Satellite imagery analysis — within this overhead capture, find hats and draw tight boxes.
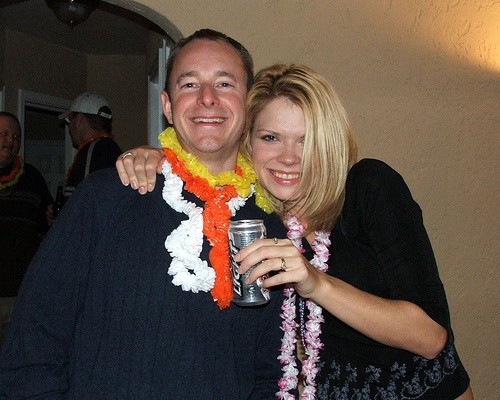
[59,90,112,121]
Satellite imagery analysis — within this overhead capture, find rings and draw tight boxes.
[122,152,134,160]
[280,255,287,271]
[272,237,279,245]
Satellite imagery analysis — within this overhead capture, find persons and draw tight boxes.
[3,28,316,400]
[57,90,123,203]
[1,110,55,298]
[243,62,475,400]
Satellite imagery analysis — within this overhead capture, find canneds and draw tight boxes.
[227,220,270,306]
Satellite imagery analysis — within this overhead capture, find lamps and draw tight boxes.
[44,0,99,27]
